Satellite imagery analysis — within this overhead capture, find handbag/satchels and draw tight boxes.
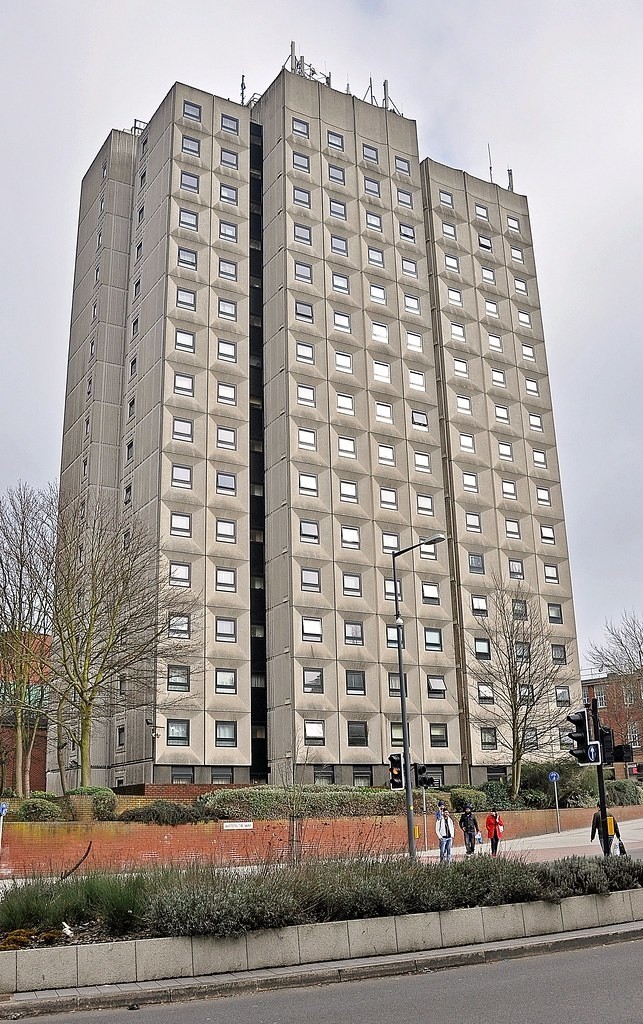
[619,838,626,855]
[475,831,482,843]
[499,826,504,832]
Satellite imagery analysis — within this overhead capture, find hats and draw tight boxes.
[439,802,444,807]
[465,803,471,808]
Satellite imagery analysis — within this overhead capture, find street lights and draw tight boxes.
[392,531,446,863]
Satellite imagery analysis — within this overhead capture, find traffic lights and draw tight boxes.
[389,753,404,791]
[417,765,434,788]
[565,709,592,766]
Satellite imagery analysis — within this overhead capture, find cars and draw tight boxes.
[637,762,643,781]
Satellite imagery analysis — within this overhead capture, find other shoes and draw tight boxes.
[466,850,474,854]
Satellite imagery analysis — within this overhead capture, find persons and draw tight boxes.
[435,809,454,864]
[459,804,479,855]
[590,801,621,855]
[485,809,504,856]
